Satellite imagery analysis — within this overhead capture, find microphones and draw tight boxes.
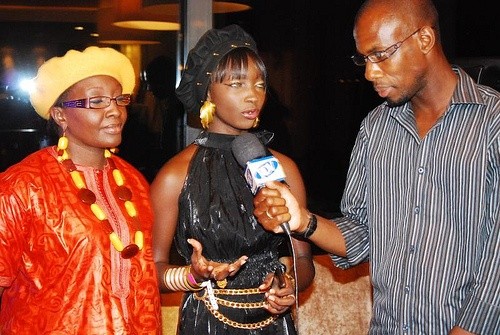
[231,133,291,234]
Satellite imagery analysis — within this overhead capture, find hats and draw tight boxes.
[28,46,135,120]
[176,24,259,116]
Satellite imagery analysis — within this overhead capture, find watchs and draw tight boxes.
[290,213,317,241]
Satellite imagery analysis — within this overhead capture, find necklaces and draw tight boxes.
[54,144,143,259]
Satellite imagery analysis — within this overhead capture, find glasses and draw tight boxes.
[57,94,131,110]
[352,27,437,66]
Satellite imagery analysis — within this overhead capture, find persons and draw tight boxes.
[0,46,163,335]
[252,0,500,335]
[148,25,315,335]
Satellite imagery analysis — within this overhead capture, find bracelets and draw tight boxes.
[162,264,206,293]
[285,270,296,295]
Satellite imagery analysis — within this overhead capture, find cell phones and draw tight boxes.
[269,268,286,298]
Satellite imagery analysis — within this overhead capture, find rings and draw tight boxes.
[214,274,227,288]
[288,294,295,299]
[265,209,273,219]
